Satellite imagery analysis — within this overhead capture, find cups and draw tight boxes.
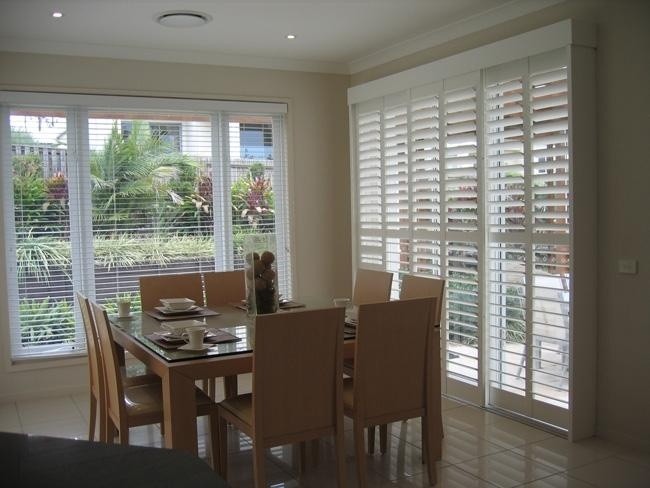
[117,298,130,316]
[181,326,205,348]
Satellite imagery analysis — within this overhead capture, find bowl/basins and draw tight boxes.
[160,319,208,335]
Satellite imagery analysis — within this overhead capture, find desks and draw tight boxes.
[102,298,359,458]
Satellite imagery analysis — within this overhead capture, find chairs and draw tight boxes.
[204,267,249,409]
[138,270,208,396]
[90,301,219,483]
[343,275,445,454]
[214,304,347,486]
[311,294,440,488]
[76,289,163,442]
[340,266,393,305]
[511,283,569,391]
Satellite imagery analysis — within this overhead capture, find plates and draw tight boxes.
[152,329,217,342]
[178,342,214,350]
[159,297,196,309]
[153,304,204,314]
